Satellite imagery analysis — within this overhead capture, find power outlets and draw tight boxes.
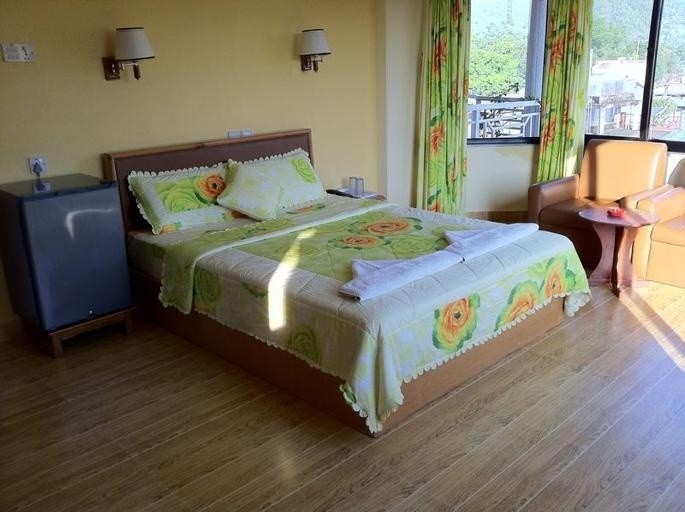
[27,153,46,173]
[2,44,36,64]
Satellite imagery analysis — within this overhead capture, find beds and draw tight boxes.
[103,129,591,438]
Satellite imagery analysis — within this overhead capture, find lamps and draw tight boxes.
[298,27,332,72]
[102,27,155,80]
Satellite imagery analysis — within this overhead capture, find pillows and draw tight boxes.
[218,164,283,221]
[227,147,327,211]
[128,163,227,235]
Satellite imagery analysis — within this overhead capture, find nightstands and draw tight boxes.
[0,174,133,355]
[327,186,384,200]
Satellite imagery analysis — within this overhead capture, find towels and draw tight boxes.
[443,222,539,263]
[337,249,464,303]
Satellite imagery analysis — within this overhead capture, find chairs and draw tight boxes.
[528,139,668,268]
[635,157,685,288]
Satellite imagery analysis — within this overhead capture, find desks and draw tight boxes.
[579,206,660,298]
